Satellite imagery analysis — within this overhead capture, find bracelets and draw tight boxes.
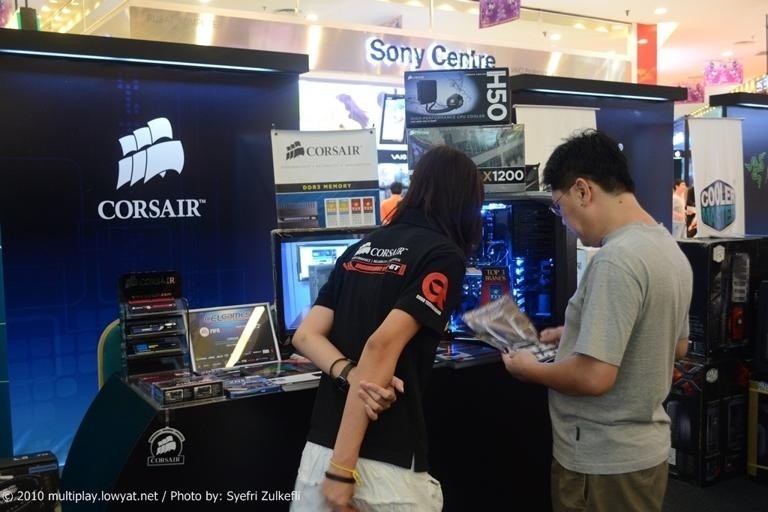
[328,354,349,383]
[324,472,357,485]
[329,459,363,487]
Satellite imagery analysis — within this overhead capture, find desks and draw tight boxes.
[435,336,553,511]
[117,358,446,512]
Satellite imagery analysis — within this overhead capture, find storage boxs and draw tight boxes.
[0,451,58,512]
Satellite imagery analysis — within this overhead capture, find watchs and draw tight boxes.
[335,360,355,390]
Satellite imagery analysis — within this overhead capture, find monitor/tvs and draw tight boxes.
[270,225,382,346]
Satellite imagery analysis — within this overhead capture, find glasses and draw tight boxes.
[548,188,574,215]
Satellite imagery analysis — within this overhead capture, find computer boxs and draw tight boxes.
[446,191,580,341]
[662,235,768,489]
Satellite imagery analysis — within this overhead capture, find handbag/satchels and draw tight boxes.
[288,441,444,511]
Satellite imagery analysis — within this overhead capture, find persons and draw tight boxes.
[673,179,688,240]
[685,186,697,238]
[381,181,404,225]
[288,146,485,511]
[502,128,694,512]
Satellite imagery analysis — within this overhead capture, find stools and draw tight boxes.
[746,387,768,477]
